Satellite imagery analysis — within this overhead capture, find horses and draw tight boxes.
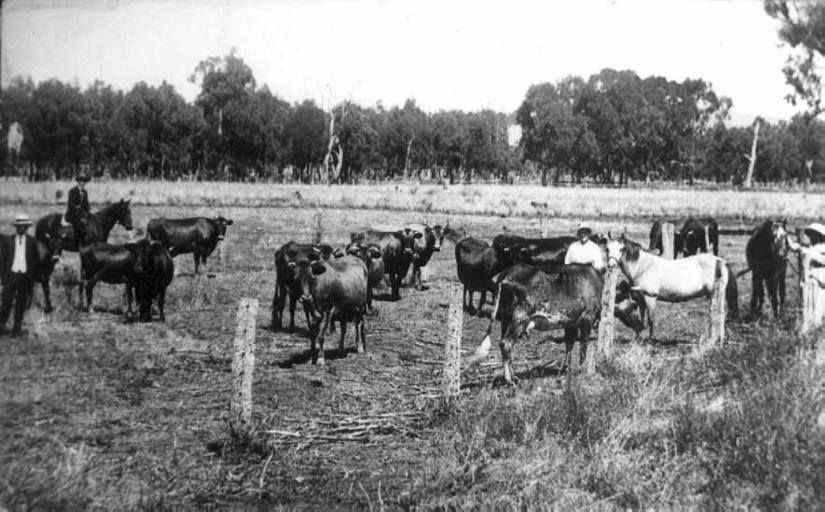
[744,216,791,316]
[602,231,740,342]
[34,197,135,269]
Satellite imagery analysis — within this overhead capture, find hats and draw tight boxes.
[805,223,825,241]
[75,173,90,181]
[13,215,32,225]
[577,223,592,235]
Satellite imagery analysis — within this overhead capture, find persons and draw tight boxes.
[0,214,39,337]
[787,222,825,330]
[64,172,90,236]
[564,228,601,270]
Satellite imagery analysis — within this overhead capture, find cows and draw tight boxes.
[1,230,71,312]
[493,229,605,264]
[453,229,518,315]
[483,261,647,386]
[144,215,234,273]
[648,215,694,256]
[682,215,720,256]
[268,218,446,329]
[288,252,377,366]
[123,235,173,325]
[72,223,176,314]
